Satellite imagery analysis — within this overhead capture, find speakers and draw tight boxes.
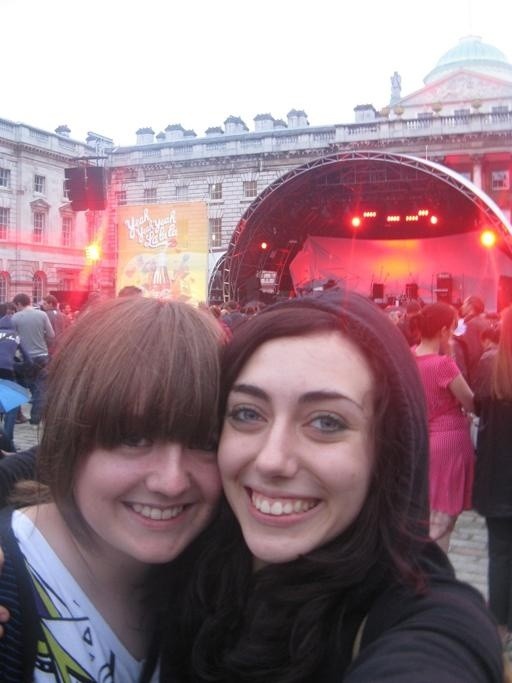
[86,168,108,211]
[63,167,88,212]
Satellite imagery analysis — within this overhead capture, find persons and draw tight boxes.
[0,281,512,683]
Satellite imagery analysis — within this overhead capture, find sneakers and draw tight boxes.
[14,411,41,428]
[1,437,16,454]
